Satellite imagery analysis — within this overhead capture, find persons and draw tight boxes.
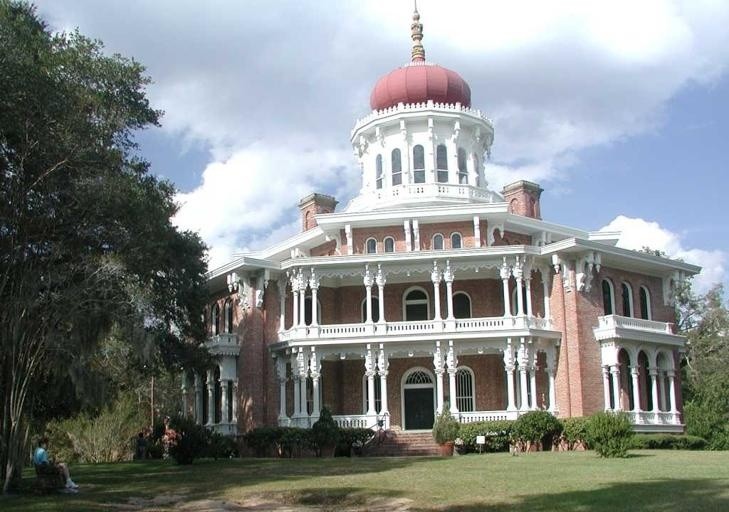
[135,432,152,458]
[33,436,79,495]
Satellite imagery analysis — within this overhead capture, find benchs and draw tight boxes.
[34,462,64,492]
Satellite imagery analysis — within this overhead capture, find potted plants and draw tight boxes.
[247,427,275,458]
[313,404,340,457]
[432,401,459,457]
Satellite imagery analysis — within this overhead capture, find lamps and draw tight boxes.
[340,353,346,360]
[408,351,414,357]
[478,347,483,354]
